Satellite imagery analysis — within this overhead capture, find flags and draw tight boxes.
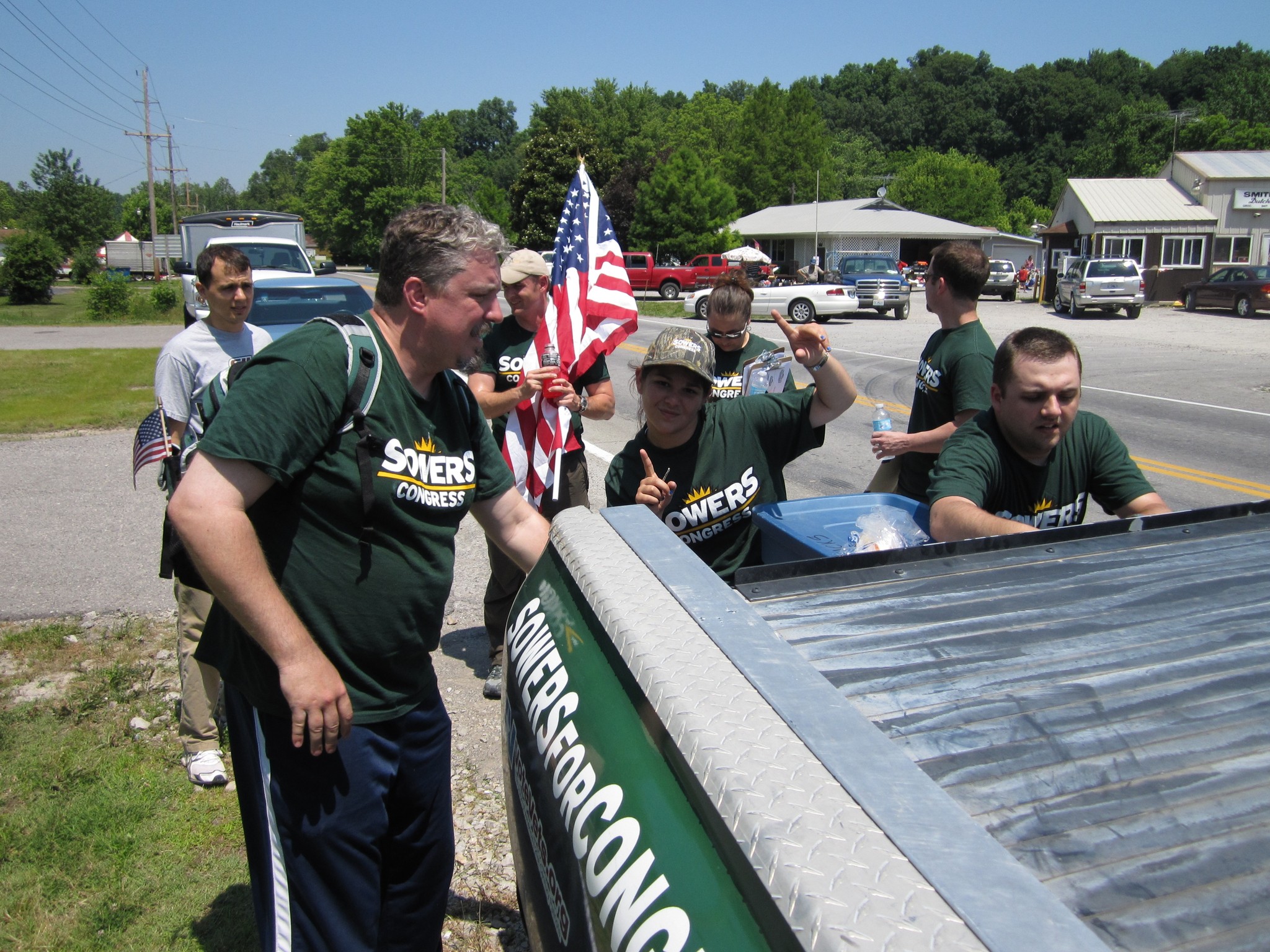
[755,240,762,251]
[501,162,639,514]
[133,407,172,491]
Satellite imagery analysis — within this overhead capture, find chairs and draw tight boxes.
[877,265,887,270]
[1233,275,1245,281]
[846,265,856,272]
[274,252,291,265]
[770,279,790,287]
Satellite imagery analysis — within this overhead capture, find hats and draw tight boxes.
[641,326,716,384]
[810,259,816,264]
[500,248,549,284]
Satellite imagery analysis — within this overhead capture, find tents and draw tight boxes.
[96,231,140,264]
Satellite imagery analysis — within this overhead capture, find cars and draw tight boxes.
[1176,265,1270,319]
[247,278,374,341]
[684,274,859,324]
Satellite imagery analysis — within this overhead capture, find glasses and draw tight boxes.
[706,319,748,338]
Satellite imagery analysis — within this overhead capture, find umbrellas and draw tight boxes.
[721,245,772,268]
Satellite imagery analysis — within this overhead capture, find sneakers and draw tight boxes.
[482,666,503,695]
[184,748,227,784]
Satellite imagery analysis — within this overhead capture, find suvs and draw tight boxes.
[539,251,556,280]
[981,259,1019,301]
[1052,254,1145,319]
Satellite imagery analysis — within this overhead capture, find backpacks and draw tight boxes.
[160,313,384,593]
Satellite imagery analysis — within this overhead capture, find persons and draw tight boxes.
[155,243,274,787]
[896,259,908,275]
[925,327,1174,542]
[863,239,997,503]
[604,310,857,584]
[169,203,552,952]
[703,269,797,402]
[797,259,831,283]
[468,249,615,698]
[1018,255,1034,293]
[741,260,764,275]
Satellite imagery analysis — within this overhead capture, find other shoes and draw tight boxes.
[1016,290,1019,293]
[1024,291,1028,293]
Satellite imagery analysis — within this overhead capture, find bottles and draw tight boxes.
[747,371,769,396]
[541,343,563,398]
[872,403,895,460]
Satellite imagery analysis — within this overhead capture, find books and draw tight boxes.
[742,347,792,396]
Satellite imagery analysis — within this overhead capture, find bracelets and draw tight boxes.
[803,352,829,372]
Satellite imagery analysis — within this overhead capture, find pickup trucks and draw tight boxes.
[504,501,1270,952]
[622,252,697,300]
[824,255,911,319]
[680,254,770,292]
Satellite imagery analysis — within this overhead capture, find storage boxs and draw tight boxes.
[751,492,931,565]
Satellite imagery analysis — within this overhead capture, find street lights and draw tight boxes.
[137,208,144,281]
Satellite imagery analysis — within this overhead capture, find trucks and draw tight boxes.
[174,210,337,329]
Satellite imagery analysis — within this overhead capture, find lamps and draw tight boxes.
[1190,178,1204,196]
[1031,219,1048,233]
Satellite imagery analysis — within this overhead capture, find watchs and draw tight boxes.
[579,395,588,414]
